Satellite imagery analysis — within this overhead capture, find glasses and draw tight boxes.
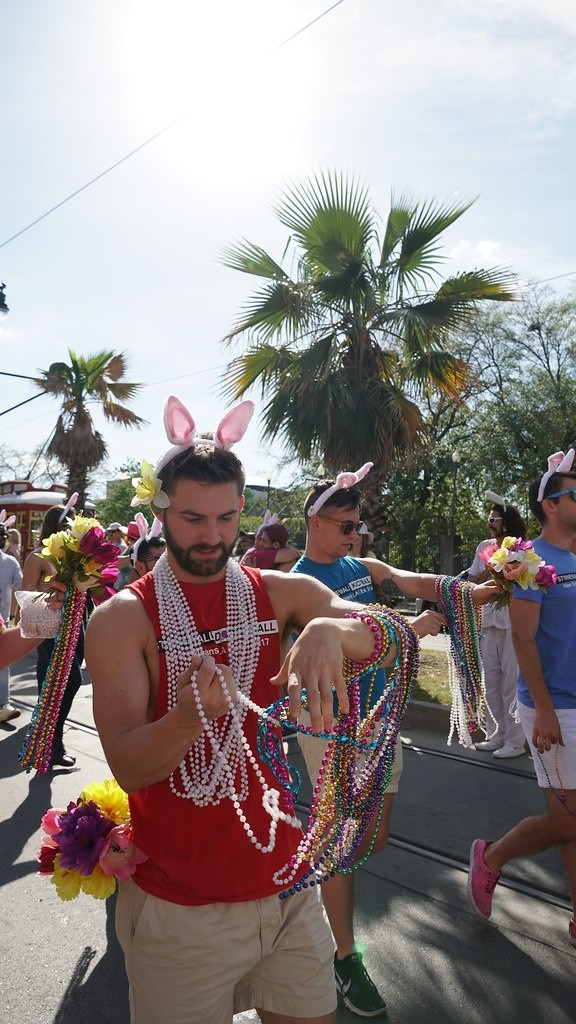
[487,517,503,525]
[546,489,576,501]
[319,516,364,535]
[144,555,161,561]
[108,530,116,533]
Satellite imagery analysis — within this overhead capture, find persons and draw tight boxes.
[276,480,504,1017]
[0,505,377,764]
[467,448,576,945]
[83,437,406,1024]
[465,488,526,758]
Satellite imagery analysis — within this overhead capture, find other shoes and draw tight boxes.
[51,754,75,766]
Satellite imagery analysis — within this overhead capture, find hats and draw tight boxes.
[266,525,288,542]
[127,524,140,541]
[106,522,122,530]
[358,521,374,545]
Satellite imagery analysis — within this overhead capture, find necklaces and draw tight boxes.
[434,574,520,749]
[17,586,87,775]
[193,603,419,896]
[537,740,566,805]
[155,546,259,804]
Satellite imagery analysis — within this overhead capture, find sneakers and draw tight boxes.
[0,704,21,724]
[475,741,502,750]
[334,950,388,1017]
[568,919,576,946]
[468,839,502,919]
[494,747,526,758]
